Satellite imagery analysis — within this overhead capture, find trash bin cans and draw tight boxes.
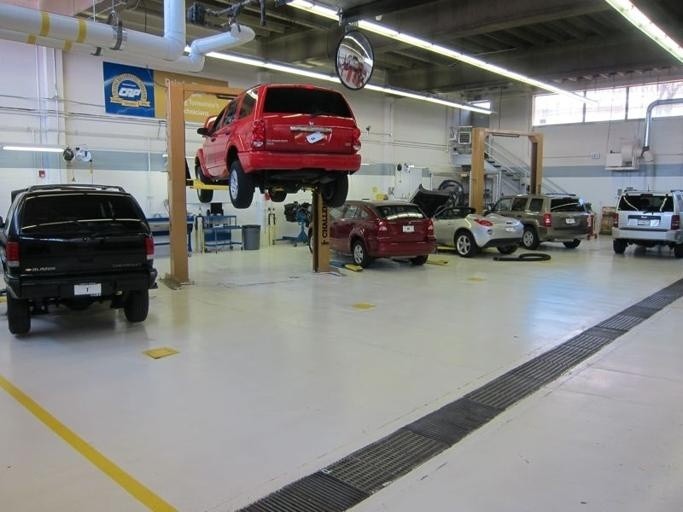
[242,224,261,250]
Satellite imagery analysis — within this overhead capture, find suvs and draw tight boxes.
[487,193,595,250]
[610,188,682,258]
[1,182,159,335]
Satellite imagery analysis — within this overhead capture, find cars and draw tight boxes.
[429,203,525,258]
[191,82,361,210]
[307,200,438,269]
